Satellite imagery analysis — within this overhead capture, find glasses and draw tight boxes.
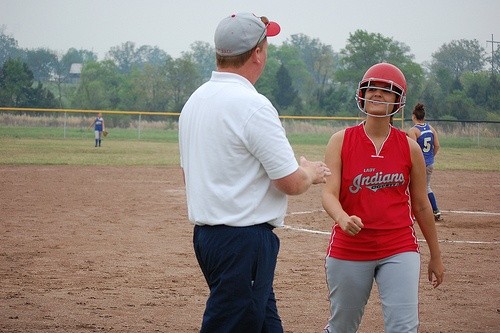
[256,16,270,47]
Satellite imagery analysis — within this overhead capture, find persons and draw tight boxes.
[407,104,441,222]
[176,10,333,333]
[320,62,446,333]
[89,111,105,147]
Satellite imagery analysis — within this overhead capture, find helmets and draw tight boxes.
[354,63,406,117]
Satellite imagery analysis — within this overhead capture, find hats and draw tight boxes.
[214,12,280,56]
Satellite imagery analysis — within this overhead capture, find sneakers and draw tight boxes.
[434,209,441,220]
